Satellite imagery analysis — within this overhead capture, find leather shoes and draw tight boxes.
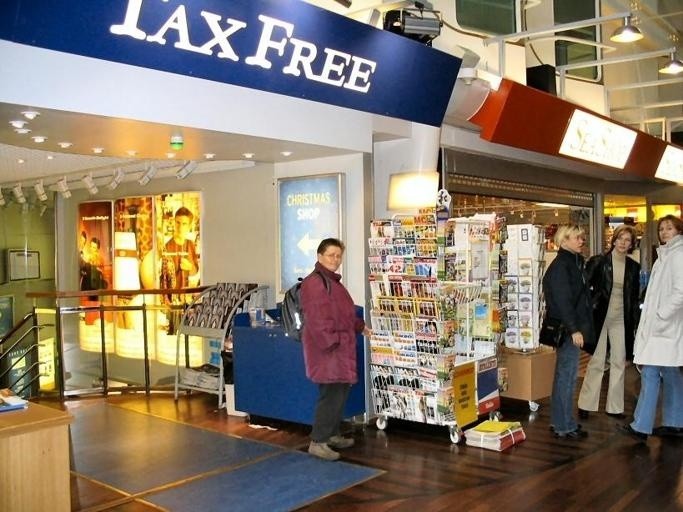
[605,412,627,421]
[653,425,682,436]
[549,423,591,442]
[615,423,648,443]
[576,407,590,420]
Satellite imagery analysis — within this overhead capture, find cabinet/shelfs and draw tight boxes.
[167,278,275,412]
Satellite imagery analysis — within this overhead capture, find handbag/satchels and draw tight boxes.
[539,282,585,351]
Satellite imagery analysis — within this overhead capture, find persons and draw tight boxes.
[614,214,683,443]
[543,225,596,440]
[578,225,641,420]
[159,207,200,321]
[80,231,104,273]
[300,238,373,462]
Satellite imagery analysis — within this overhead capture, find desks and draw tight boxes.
[230,303,366,433]
[0,386,75,511]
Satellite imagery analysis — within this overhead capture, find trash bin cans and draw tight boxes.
[221,350,248,417]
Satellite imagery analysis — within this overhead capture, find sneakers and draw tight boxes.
[326,434,355,450]
[306,441,341,462]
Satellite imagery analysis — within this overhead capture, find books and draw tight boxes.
[368,207,491,424]
[464,419,526,452]
[0,388,28,412]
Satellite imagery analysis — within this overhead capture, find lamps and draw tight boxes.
[451,194,559,222]
[0,156,197,210]
[555,45,683,104]
[385,168,441,211]
[479,9,647,82]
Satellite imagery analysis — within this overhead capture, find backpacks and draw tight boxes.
[279,269,331,344]
